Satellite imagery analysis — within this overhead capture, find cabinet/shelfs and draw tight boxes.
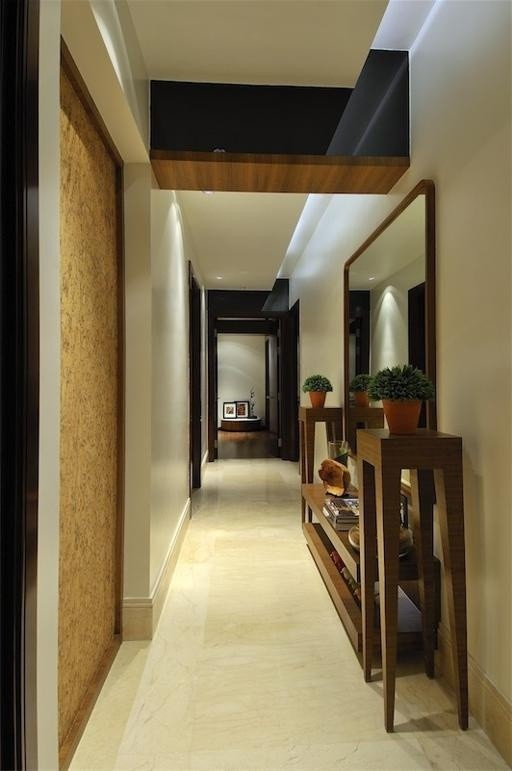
[220,418,263,431]
[345,405,382,455]
[301,478,442,659]
[349,421,472,732]
[298,407,344,532]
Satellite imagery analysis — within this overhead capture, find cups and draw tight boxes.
[327,441,349,469]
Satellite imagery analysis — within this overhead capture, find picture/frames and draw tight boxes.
[234,401,251,419]
[222,402,238,420]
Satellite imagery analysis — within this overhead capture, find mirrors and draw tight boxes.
[342,179,438,460]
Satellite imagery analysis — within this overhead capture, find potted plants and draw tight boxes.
[349,373,374,407]
[301,375,334,410]
[368,363,435,439]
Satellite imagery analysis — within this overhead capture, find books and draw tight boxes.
[320,496,360,532]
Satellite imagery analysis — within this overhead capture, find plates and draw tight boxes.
[348,524,414,562]
